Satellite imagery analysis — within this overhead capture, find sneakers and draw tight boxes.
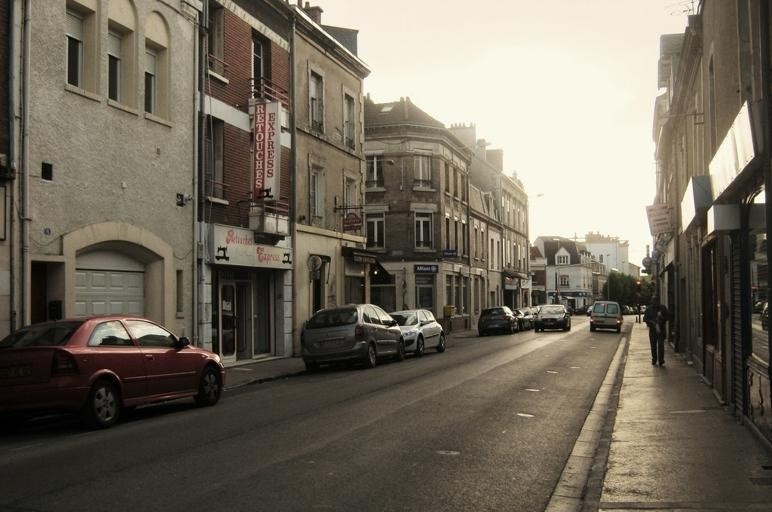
[651,358,666,366]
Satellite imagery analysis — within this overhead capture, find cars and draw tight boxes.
[622,305,646,314]
[478,305,573,337]
[0,314,226,429]
[301,303,446,374]
[587,306,594,316]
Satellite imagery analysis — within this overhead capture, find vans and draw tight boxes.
[590,300,623,333]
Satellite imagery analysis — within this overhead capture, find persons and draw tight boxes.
[643,294,671,368]
